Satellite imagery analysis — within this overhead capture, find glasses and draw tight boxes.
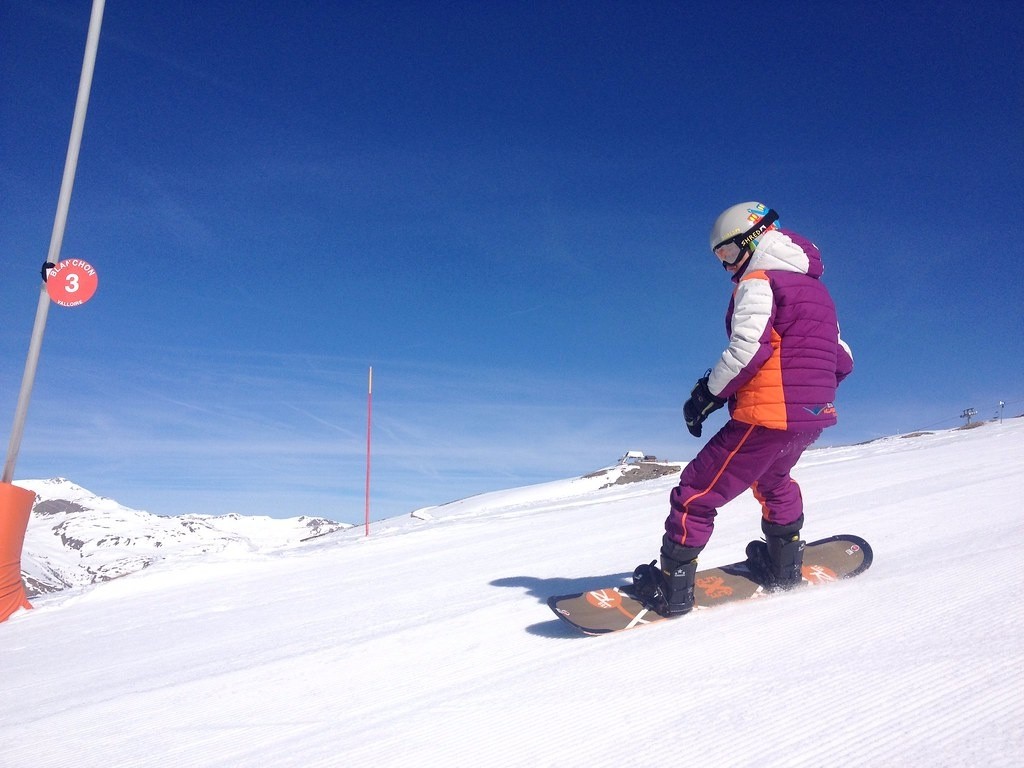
[713,209,779,271]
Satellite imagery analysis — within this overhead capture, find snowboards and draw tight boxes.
[549,534,874,634]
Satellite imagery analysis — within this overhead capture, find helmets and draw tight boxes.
[710,202,781,252]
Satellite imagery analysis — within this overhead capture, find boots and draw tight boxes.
[633,555,698,617]
[746,530,817,591]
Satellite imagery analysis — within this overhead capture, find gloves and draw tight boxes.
[683,368,728,437]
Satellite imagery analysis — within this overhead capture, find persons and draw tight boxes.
[634,201,854,616]
